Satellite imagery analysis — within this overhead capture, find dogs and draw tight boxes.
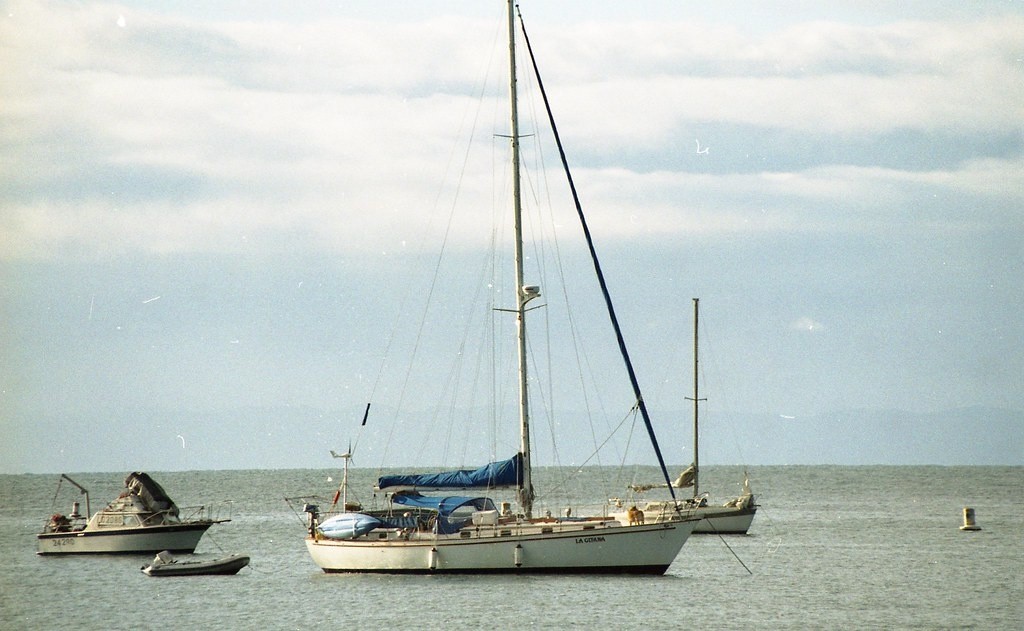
[627,506,645,526]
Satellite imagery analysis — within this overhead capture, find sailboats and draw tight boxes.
[606,297,761,535]
[284,0,707,576]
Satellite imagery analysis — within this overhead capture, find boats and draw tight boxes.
[34,470,237,556]
[139,546,250,578]
